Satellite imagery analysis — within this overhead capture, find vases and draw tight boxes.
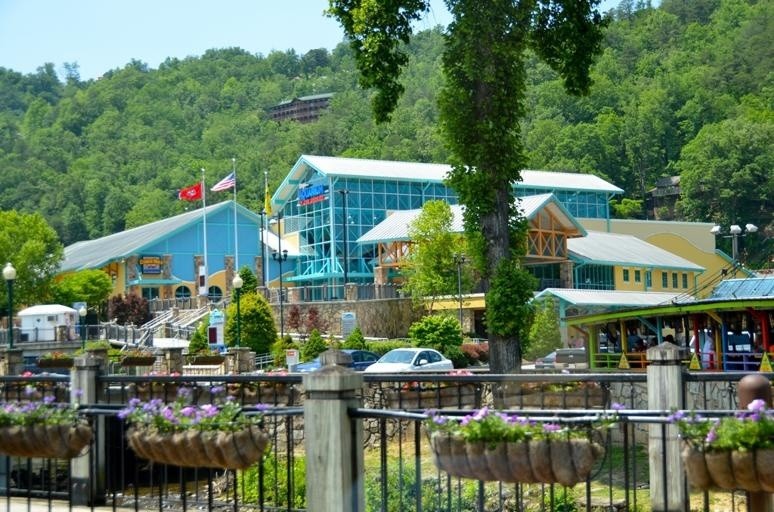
[427,431,608,489]
[126,427,271,469]
[678,434,774,493]
[0,424,95,459]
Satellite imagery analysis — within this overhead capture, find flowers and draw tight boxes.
[0,384,84,425]
[420,399,631,440]
[665,399,774,451]
[117,384,267,431]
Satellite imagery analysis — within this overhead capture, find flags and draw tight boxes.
[210,171,235,192]
[265,181,273,217]
[179,182,202,200]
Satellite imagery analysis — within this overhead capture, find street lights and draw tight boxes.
[271,208,289,338]
[78,306,86,352]
[232,274,243,348]
[452,251,466,338]
[338,187,352,288]
[2,262,16,348]
[710,222,758,279]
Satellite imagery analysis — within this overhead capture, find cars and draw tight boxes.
[364,347,453,390]
[535,329,757,370]
[294,348,379,377]
